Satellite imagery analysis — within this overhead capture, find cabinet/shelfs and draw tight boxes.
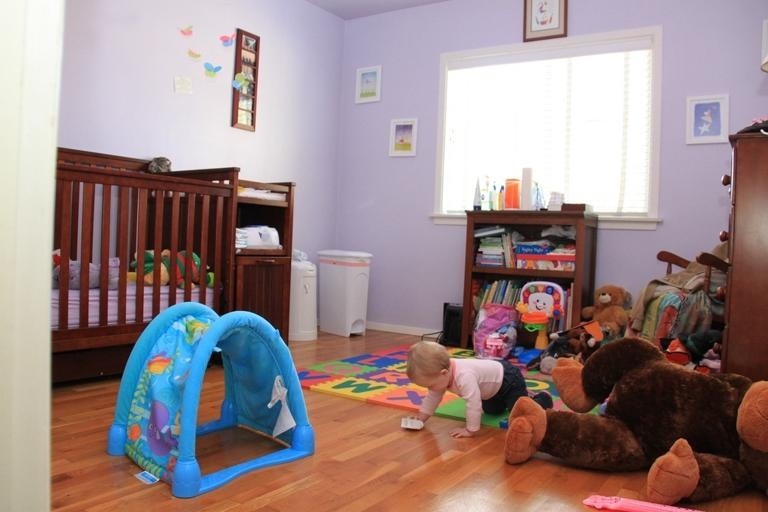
[460,209,598,349]
[161,167,296,346]
[717,130,768,383]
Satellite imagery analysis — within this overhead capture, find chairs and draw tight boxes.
[656,248,729,365]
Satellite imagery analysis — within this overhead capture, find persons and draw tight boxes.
[406,341,551,438]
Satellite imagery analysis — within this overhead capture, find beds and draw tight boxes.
[49,146,240,356]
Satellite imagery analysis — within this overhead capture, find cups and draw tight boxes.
[504,179,521,209]
[521,167,533,211]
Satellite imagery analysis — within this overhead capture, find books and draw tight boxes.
[472,228,573,329]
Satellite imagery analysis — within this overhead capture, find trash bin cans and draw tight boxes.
[288,249,374,342]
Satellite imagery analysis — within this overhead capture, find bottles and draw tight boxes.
[473,175,504,210]
[533,183,546,210]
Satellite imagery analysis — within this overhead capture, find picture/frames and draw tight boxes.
[232,27,260,132]
[523,0,568,42]
[685,94,729,144]
[354,64,381,104]
[388,118,417,157]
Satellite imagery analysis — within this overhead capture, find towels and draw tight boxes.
[234,223,280,249]
